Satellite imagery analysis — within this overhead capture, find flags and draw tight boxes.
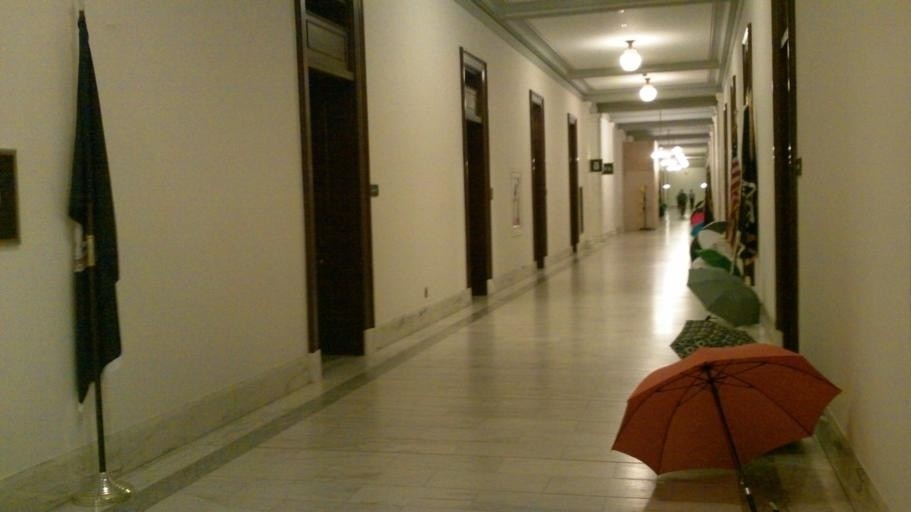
[62,12,128,402]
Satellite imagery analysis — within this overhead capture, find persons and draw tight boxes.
[688,190,695,209]
[676,189,688,217]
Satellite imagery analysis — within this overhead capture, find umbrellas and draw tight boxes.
[685,267,762,328]
[609,341,845,512]
[690,200,746,280]
[669,319,755,366]
